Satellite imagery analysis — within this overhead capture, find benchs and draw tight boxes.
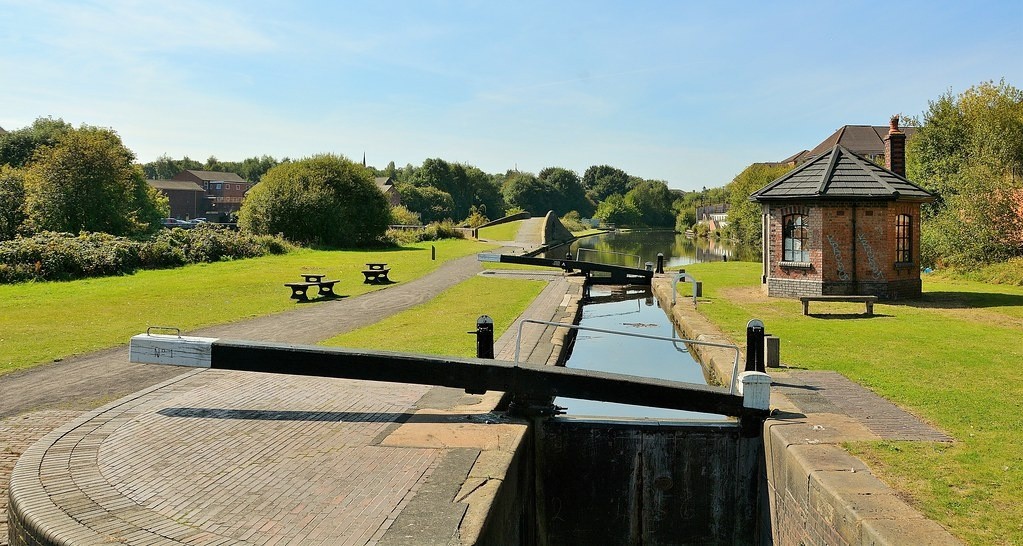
[283,280,340,301]
[361,267,391,284]
[797,295,879,316]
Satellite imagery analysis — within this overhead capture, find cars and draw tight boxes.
[161,218,206,229]
